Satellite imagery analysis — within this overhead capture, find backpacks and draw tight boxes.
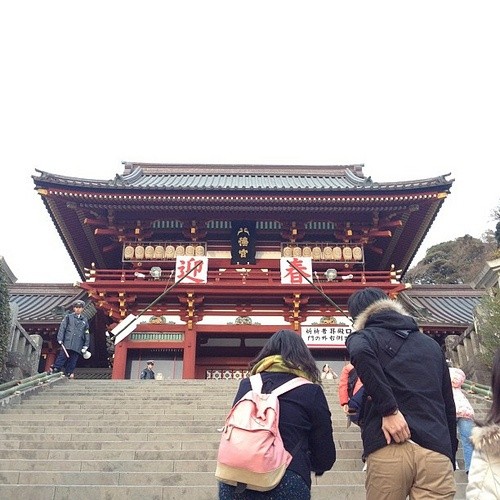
[214,372,315,491]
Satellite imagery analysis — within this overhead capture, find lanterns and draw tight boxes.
[135,246,144,259]
[303,246,321,261]
[343,247,362,261]
[186,246,204,256]
[125,246,134,259]
[155,246,164,259]
[165,245,185,260]
[323,246,343,261]
[145,246,154,259]
[283,247,301,257]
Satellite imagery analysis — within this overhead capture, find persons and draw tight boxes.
[340,362,363,413]
[345,287,459,500]
[448,367,477,474]
[465,346,500,500]
[220,329,336,500]
[49,299,90,379]
[140,362,154,379]
[320,364,338,379]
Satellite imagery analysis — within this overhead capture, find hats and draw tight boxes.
[147,360,154,365]
[73,299,85,307]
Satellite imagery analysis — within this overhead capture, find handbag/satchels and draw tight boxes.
[346,383,376,427]
